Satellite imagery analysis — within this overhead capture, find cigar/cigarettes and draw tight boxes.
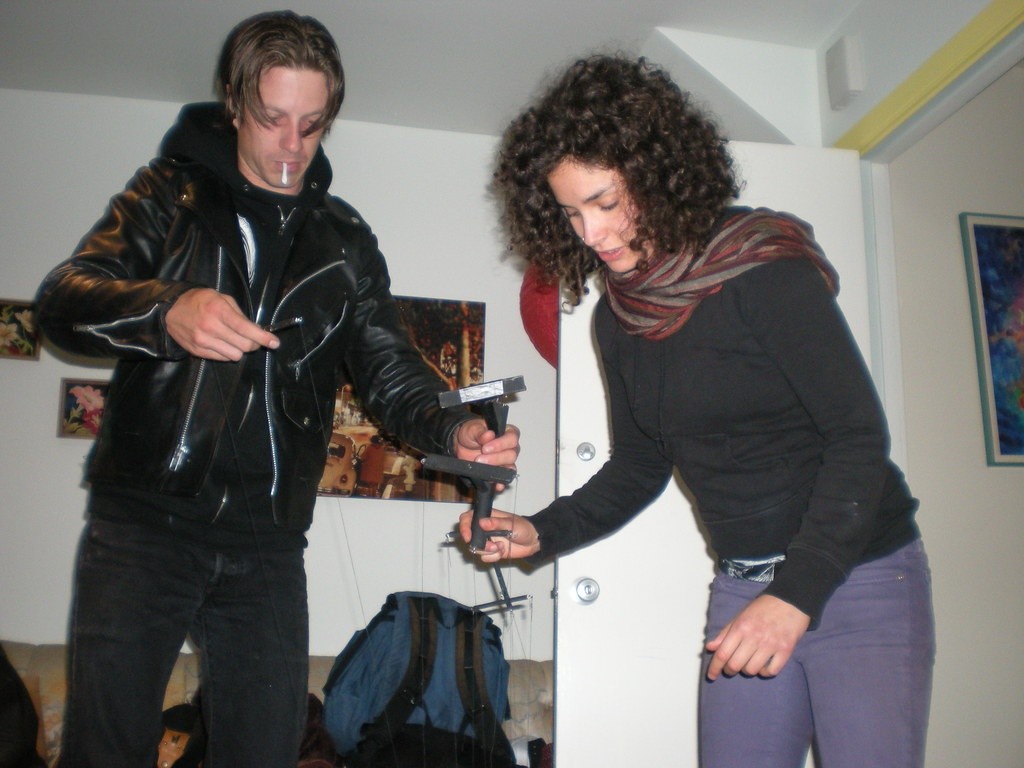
[282,162,288,184]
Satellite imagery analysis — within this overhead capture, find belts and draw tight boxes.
[717,556,782,583]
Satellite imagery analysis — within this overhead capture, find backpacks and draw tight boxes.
[321,592,517,768]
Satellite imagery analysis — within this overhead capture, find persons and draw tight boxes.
[33,6,520,768]
[459,48,935,768]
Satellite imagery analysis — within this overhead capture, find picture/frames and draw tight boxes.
[56,376,110,440]
[0,298,42,361]
[957,211,1024,467]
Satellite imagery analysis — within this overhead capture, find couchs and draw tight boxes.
[0,640,553,768]
